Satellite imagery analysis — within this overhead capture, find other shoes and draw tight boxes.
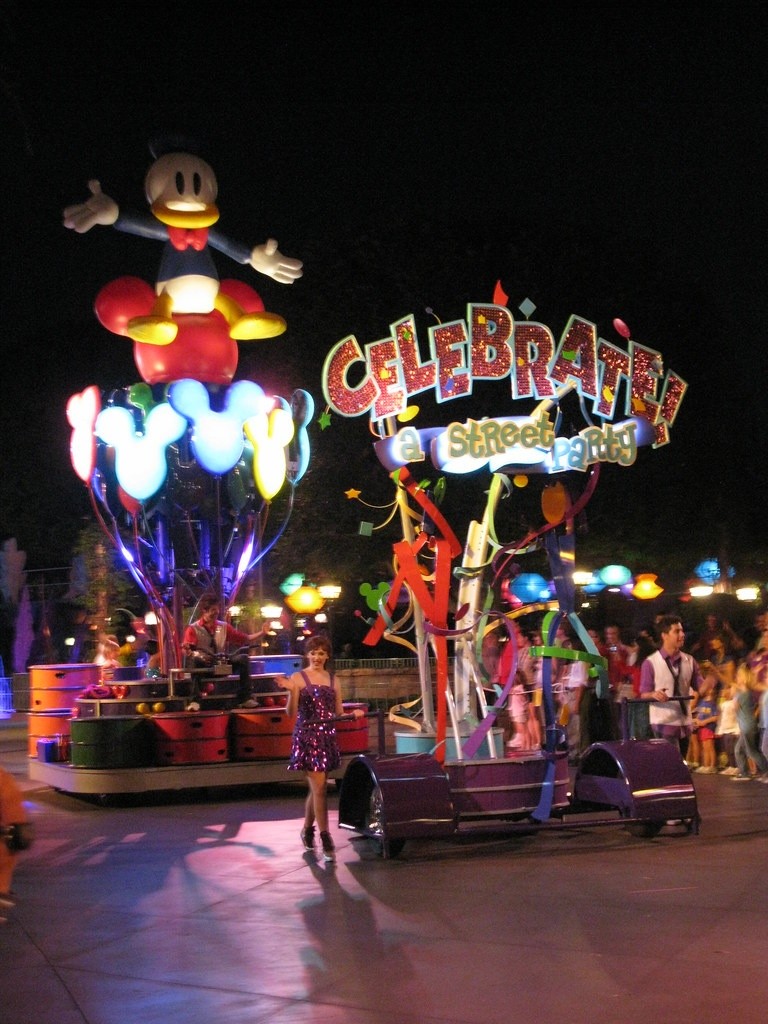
[319,831,335,855]
[300,826,317,850]
[761,776,768,784]
[694,766,717,774]
[188,702,200,711]
[730,775,753,780]
[666,819,681,825]
[720,767,741,775]
[238,700,260,708]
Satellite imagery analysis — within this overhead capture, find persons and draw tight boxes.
[274,636,364,860]
[145,640,161,677]
[181,593,269,711]
[482,613,768,784]
[93,635,120,668]
[0,770,25,920]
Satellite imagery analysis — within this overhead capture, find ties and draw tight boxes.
[204,625,218,654]
[666,657,687,716]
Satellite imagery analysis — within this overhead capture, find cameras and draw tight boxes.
[609,646,618,652]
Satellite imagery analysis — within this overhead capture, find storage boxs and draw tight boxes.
[249,654,304,676]
